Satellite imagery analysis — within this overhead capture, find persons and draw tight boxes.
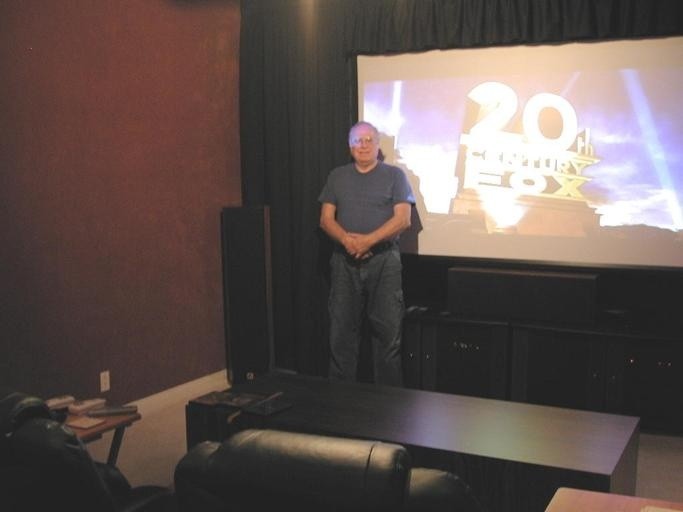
[318,120,418,383]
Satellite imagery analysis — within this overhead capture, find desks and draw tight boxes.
[185,371,641,512]
[542,486,682,512]
[63,405,141,466]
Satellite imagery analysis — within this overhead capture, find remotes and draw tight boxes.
[68,396,106,416]
[89,404,138,416]
[44,393,76,410]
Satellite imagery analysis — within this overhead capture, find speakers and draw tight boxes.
[219,204,275,386]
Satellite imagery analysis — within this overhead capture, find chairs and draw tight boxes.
[0,385,167,512]
[173,428,483,512]
